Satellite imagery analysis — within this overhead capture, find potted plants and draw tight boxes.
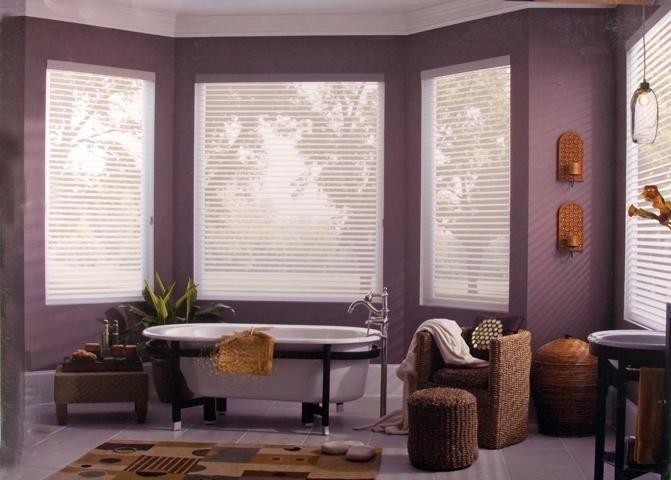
[116,272,237,404]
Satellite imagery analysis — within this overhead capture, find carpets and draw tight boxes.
[40,437,384,480]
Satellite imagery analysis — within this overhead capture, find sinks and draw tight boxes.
[586,328,666,362]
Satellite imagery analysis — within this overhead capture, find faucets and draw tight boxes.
[346,298,383,316]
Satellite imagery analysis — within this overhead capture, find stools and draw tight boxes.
[408,387,481,472]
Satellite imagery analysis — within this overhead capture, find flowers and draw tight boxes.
[627,183,671,232]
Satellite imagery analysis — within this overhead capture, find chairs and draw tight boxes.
[413,324,533,450]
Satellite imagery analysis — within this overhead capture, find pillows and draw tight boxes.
[467,311,526,362]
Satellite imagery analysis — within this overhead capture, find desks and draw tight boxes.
[586,326,671,480]
[53,361,150,426]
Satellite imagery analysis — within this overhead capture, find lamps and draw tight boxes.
[556,201,585,260]
[626,0,661,146]
[557,131,586,189]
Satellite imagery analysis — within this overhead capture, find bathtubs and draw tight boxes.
[141,322,383,402]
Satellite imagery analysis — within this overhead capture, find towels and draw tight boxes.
[635,366,668,467]
[351,317,496,437]
